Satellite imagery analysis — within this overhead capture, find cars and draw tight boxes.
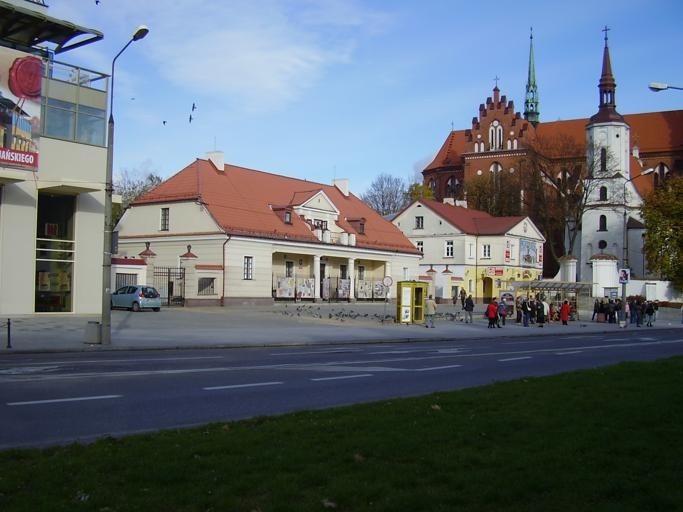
[112,285,161,313]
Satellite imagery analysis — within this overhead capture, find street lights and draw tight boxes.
[649,83,683,92]
[101,25,148,342]
[619,168,655,321]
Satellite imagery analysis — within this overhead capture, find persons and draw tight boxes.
[425,294,438,328]
[592,295,661,328]
[620,270,628,283]
[452,295,456,308]
[465,294,475,323]
[458,287,467,309]
[484,295,572,329]
